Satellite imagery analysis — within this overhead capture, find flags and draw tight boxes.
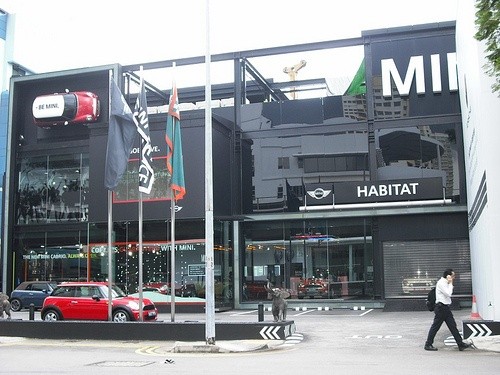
[165,82,185,202]
[133,75,155,194]
[104,76,138,191]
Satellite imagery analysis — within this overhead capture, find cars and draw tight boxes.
[33,88,101,131]
[9,281,58,312]
[135,269,293,301]
[297,283,327,299]
[41,283,158,321]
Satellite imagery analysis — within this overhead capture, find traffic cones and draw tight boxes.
[470,294,480,318]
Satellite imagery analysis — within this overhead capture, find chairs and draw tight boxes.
[71,287,82,297]
[88,288,96,296]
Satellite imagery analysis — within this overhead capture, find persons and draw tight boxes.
[19,177,90,224]
[424,268,473,351]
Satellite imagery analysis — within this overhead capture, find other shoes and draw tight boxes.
[459,343,472,351]
[424,345,438,351]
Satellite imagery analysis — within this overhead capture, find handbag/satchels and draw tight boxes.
[427,287,436,311]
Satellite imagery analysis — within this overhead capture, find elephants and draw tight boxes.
[264,278,291,323]
[0,292,11,319]
